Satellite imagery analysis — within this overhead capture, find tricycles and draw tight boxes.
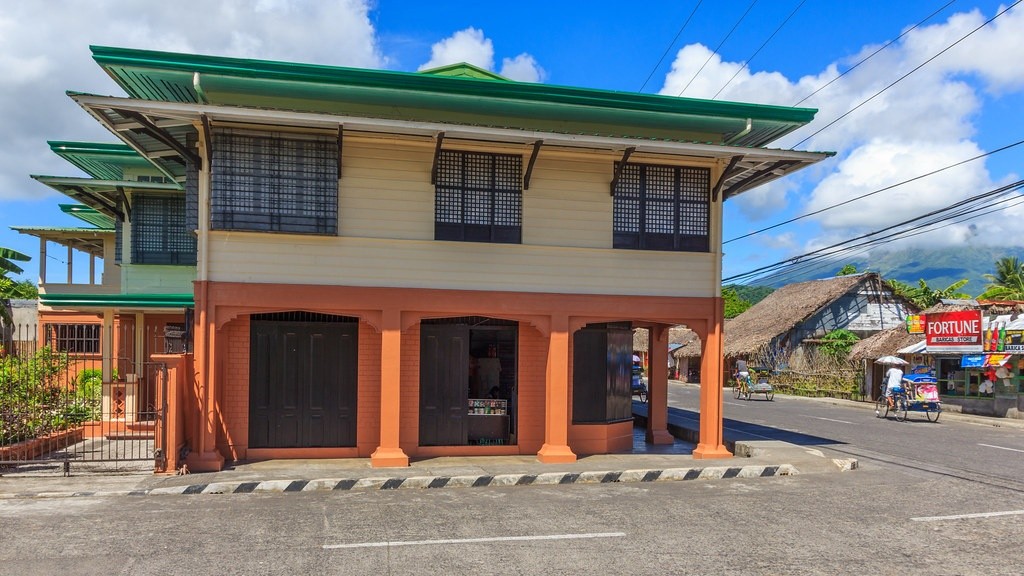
[631,366,647,403]
[732,374,774,401]
[876,373,945,422]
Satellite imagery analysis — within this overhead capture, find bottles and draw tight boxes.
[990,322,999,351]
[984,322,992,351]
[997,321,1006,351]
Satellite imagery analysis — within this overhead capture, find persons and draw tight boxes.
[886,364,903,410]
[732,357,749,389]
[633,362,644,375]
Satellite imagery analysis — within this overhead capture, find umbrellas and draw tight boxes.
[633,355,640,362]
[873,356,909,368]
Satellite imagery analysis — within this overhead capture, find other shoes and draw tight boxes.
[736,386,741,390]
[890,405,895,410]
[744,390,748,394]
